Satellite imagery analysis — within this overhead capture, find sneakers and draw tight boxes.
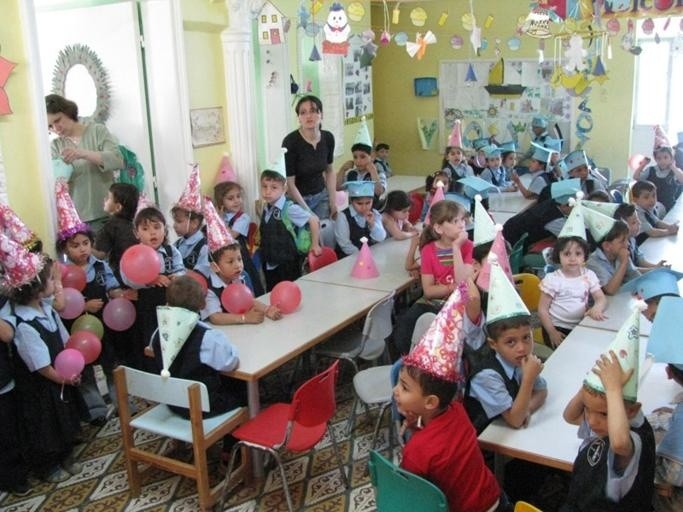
[74,425,87,443]
[12,478,36,498]
[47,462,70,483]
[218,454,239,477]
[91,414,108,426]
[61,456,84,474]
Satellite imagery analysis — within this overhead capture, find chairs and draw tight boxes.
[217,355,349,512]
[110,364,252,512]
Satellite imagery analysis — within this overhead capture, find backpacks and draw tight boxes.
[281,199,315,256]
[231,210,264,275]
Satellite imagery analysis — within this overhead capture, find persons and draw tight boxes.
[280,95,339,222]
[123,115,421,400]
[391,120,683,511]
[0,175,139,502]
[44,93,124,226]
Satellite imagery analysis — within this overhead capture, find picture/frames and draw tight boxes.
[190,105,227,150]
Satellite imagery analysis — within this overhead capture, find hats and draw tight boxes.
[1,196,47,293]
[174,153,243,254]
[402,281,471,385]
[134,191,162,227]
[351,116,373,147]
[471,195,534,325]
[585,267,683,403]
[457,114,591,204]
[424,182,450,225]
[339,181,377,198]
[348,236,380,279]
[446,119,462,148]
[155,306,199,380]
[259,147,290,180]
[54,179,88,238]
[557,190,621,242]
[651,125,672,153]
